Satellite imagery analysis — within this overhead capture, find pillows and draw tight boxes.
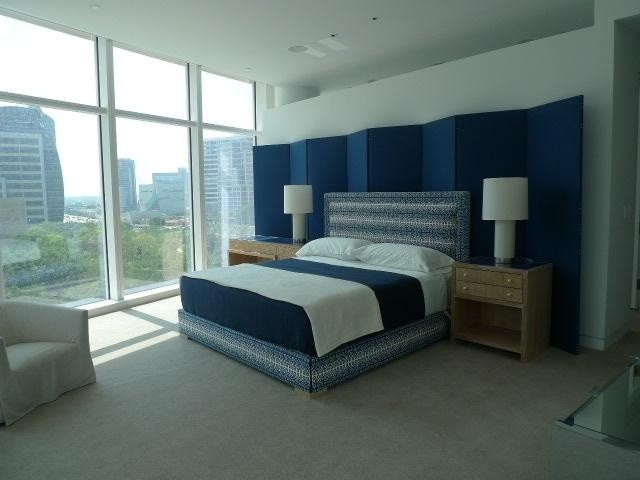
[351,242,457,272]
[294,236,373,262]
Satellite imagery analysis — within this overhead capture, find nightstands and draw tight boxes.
[226,234,302,266]
[447,254,557,363]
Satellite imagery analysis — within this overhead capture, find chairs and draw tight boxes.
[1,298,101,429]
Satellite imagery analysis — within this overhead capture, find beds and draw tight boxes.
[173,187,478,403]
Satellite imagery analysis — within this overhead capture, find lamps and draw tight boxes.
[480,176,532,266]
[281,183,316,243]
